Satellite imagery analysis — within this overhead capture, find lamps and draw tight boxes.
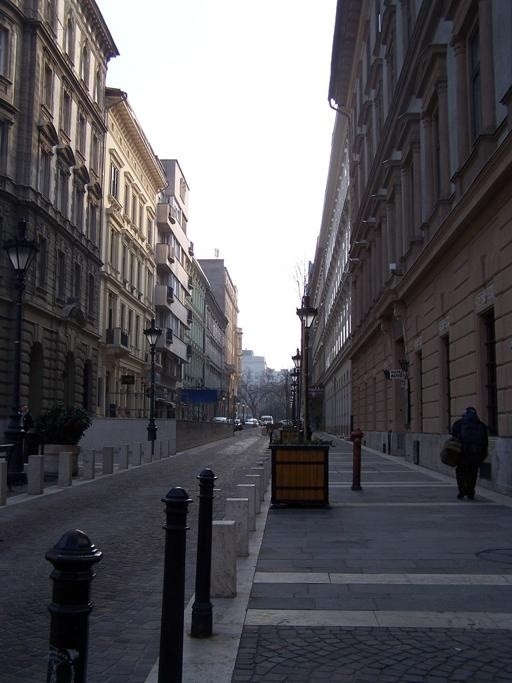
[387,261,401,276]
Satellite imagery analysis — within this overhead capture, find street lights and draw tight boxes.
[292,348,300,421]
[142,316,163,456]
[293,293,319,444]
[287,370,297,421]
[242,401,245,425]
[3,212,43,482]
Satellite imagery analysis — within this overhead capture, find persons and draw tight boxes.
[451,405,490,498]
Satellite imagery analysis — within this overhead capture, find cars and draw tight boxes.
[278,419,292,426]
[244,418,260,427]
[210,415,244,432]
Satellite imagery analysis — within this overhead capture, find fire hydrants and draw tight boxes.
[349,427,366,491]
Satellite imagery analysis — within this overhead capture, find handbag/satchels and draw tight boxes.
[479,461,493,480]
[439,437,463,467]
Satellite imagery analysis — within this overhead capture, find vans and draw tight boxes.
[260,415,274,426]
